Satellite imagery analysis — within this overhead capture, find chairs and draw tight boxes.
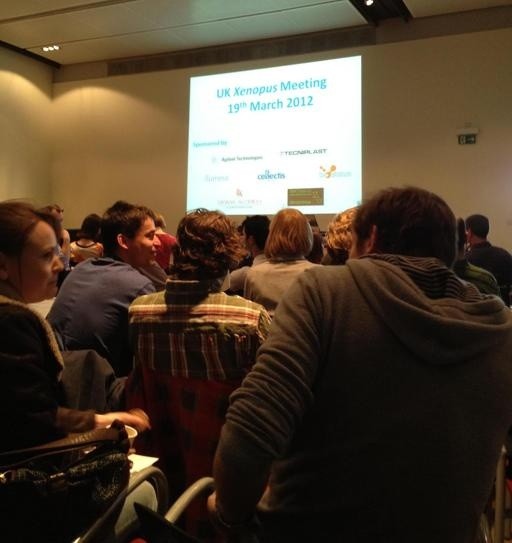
[160,444,512,541]
[134,369,263,518]
[56,346,125,412]
[70,465,170,542]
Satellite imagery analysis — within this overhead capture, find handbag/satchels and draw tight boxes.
[1,419,130,542]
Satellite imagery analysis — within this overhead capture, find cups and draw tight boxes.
[106,424,138,447]
